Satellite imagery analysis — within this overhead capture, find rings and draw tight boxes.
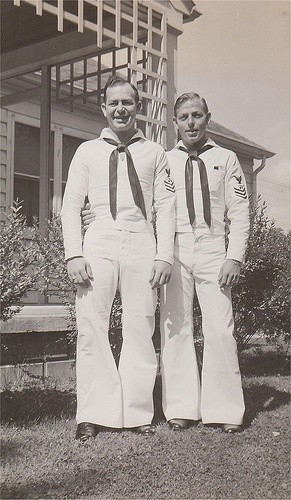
[73,276,77,280]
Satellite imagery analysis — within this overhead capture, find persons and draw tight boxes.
[61,75,177,442]
[80,92,250,433]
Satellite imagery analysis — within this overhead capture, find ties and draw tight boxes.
[103,137,148,221]
[180,145,211,228]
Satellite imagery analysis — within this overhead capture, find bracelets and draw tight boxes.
[230,260,242,268]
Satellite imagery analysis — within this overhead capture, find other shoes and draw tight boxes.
[138,425,153,434]
[169,418,190,430]
[75,422,94,440]
[222,423,240,432]
